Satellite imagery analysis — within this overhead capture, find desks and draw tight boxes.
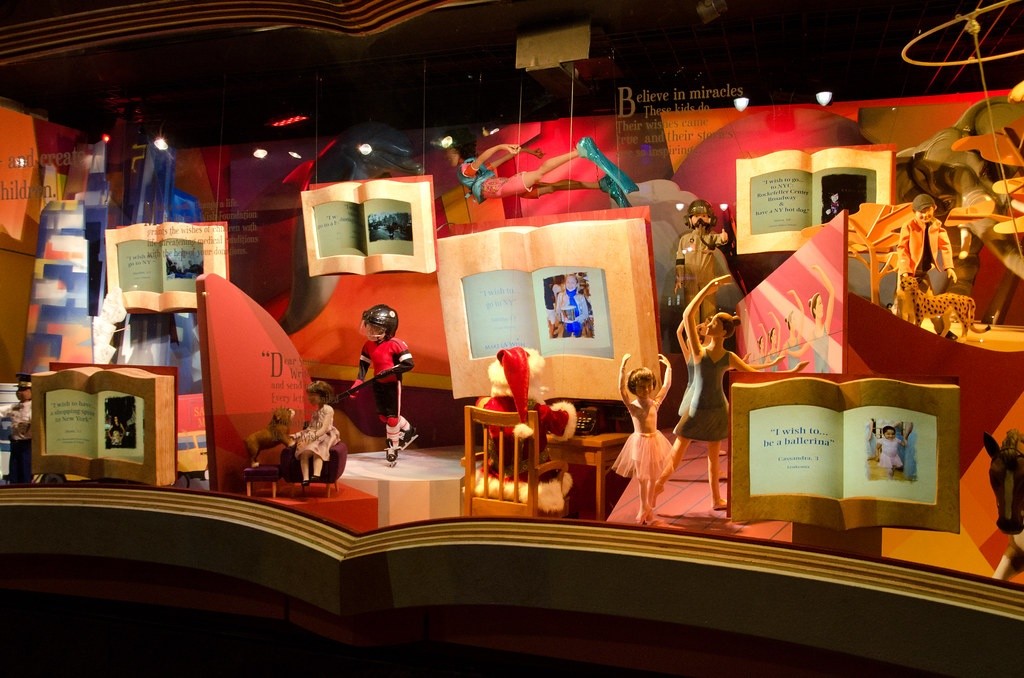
[547,430,635,520]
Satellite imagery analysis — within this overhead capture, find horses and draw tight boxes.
[983,429,1024,581]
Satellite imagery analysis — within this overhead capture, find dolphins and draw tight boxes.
[278,122,424,336]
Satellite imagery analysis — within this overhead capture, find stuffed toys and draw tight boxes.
[471,347,579,517]
[243,405,297,466]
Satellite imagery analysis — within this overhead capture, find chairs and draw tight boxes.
[282,441,348,494]
[460,404,570,518]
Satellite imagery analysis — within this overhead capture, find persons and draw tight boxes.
[349,304,418,463]
[897,195,958,339]
[612,200,808,528]
[894,475,897,481]
[0,374,32,481]
[544,273,595,339]
[869,421,919,477]
[108,414,126,446]
[289,381,341,486]
[443,128,639,204]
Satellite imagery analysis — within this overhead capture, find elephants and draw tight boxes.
[895,96,1024,297]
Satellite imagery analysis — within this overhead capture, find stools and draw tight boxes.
[244,462,282,498]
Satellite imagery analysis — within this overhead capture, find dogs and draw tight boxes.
[242,407,297,468]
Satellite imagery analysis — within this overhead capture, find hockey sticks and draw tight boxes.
[325,364,401,406]
